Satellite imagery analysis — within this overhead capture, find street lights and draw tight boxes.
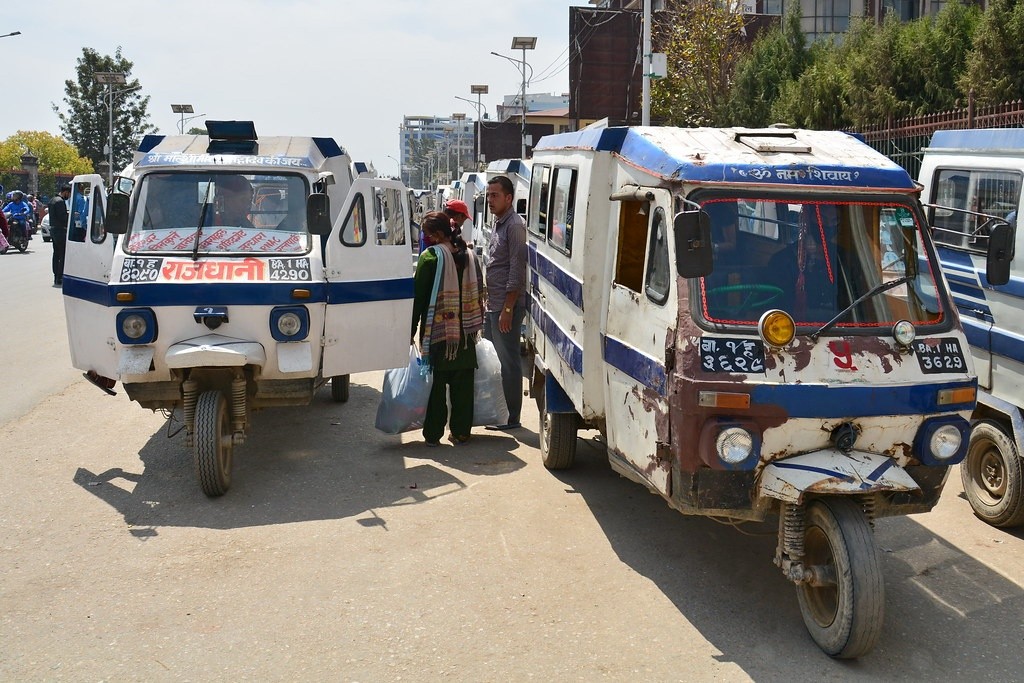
[454,96,486,172]
[104,85,142,185]
[176,113,207,134]
[388,156,399,179]
[491,51,533,159]
[415,121,465,192]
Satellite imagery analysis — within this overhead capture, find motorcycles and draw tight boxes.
[906,128,1024,528]
[524,126,1016,658]
[0,208,29,255]
[62,120,414,497]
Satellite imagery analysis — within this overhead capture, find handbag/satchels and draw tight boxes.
[445,338,509,430]
[376,342,433,434]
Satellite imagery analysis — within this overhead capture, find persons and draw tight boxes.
[411,175,528,447]
[213,177,289,229]
[708,201,737,266]
[0,184,101,287]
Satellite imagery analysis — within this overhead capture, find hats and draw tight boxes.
[445,199,473,221]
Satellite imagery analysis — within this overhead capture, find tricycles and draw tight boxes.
[378,159,533,357]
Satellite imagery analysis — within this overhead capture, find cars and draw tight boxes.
[41,195,102,242]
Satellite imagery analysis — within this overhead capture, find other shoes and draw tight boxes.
[426,437,440,446]
[29,236,34,241]
[487,423,521,429]
[449,433,466,444]
[54,274,62,285]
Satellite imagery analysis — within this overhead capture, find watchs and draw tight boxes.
[502,306,513,313]
[714,244,719,252]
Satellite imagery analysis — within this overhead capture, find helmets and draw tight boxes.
[5,190,34,202]
